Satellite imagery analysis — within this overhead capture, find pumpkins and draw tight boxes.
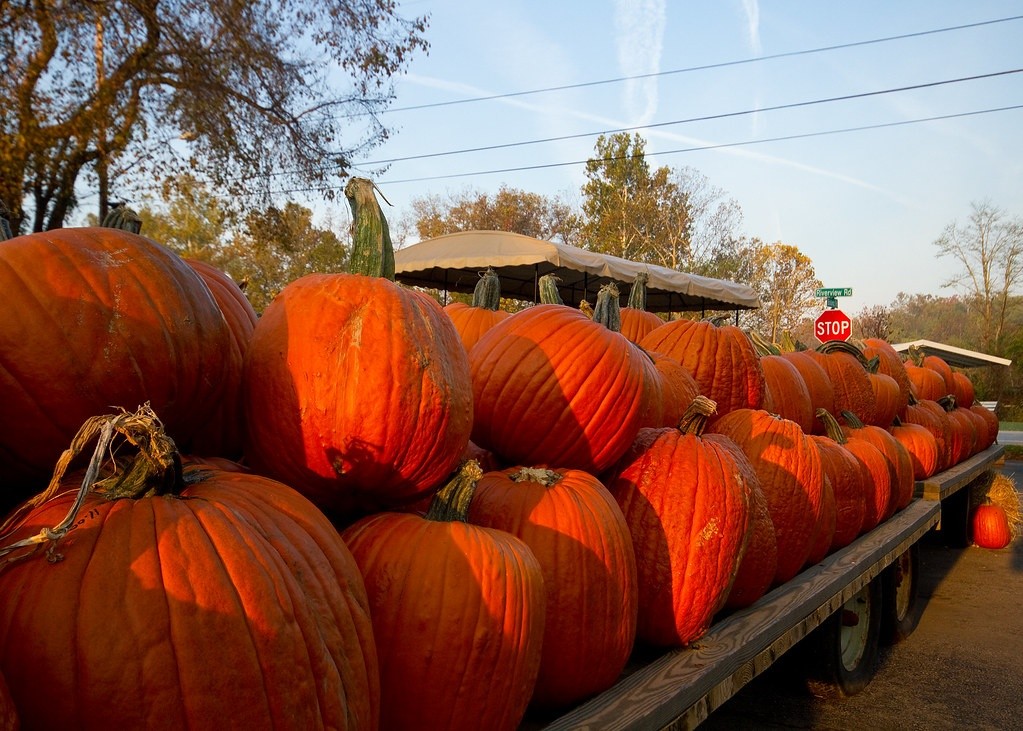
[0,179,1011,731]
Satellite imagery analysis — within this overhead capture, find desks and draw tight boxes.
[911,444,1007,530]
[539,498,942,731]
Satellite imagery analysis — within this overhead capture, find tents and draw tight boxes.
[890,338,1012,369]
[392,230,763,316]
